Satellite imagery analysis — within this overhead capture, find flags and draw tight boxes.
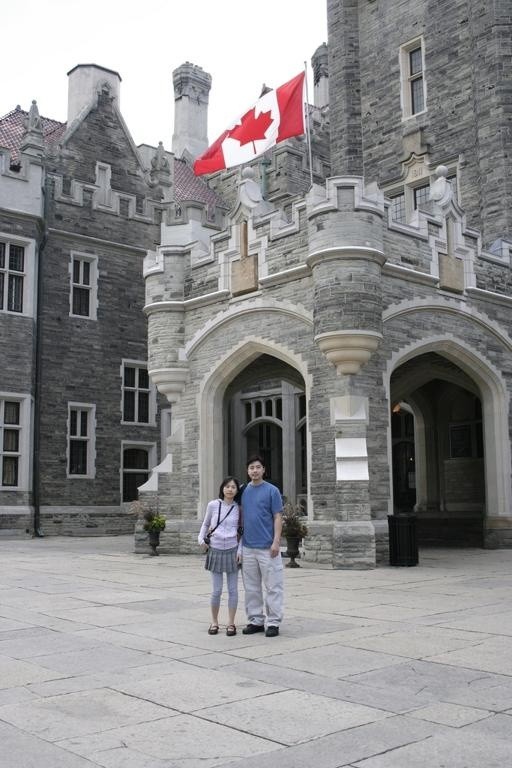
[192,73,306,176]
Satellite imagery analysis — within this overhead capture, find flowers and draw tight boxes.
[280,499,310,540]
[129,496,167,534]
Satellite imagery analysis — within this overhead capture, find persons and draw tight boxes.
[239,457,283,636]
[197,477,243,636]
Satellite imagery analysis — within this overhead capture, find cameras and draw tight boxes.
[204,536,211,544]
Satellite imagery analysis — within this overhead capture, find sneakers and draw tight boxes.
[266,626,279,638]
[243,624,265,634]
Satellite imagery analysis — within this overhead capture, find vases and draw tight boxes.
[148,535,161,557]
[285,537,302,569]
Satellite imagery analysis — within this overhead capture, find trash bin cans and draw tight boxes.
[387,516,416,566]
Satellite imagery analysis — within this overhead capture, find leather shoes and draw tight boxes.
[208,623,218,635]
[226,624,236,637]
